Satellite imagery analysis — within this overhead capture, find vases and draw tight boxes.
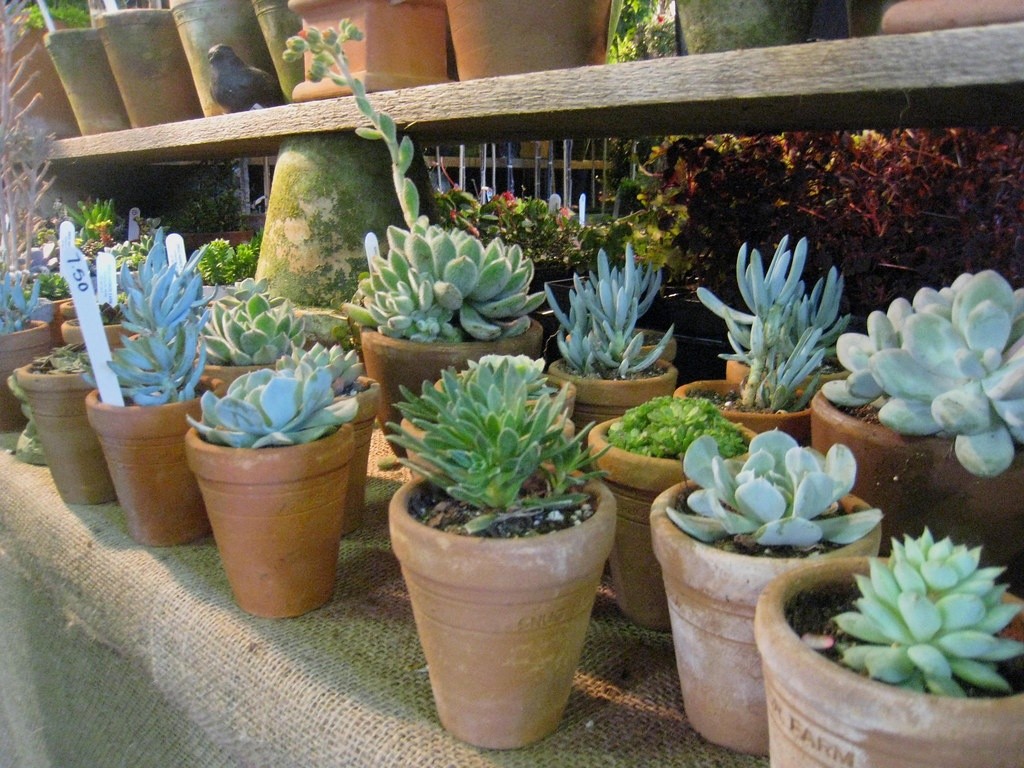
[445,0,1024,135]
[168,0,288,119]
[86,7,205,130]
[43,27,130,136]
[287,0,454,104]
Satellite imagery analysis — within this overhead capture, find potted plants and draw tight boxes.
[0,3,93,142]
[588,392,761,630]
[695,231,857,397]
[0,189,681,751]
[672,304,825,451]
[650,424,883,759]
[808,265,1024,599]
[754,517,1024,768]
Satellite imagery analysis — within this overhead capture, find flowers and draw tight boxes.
[444,187,582,265]
